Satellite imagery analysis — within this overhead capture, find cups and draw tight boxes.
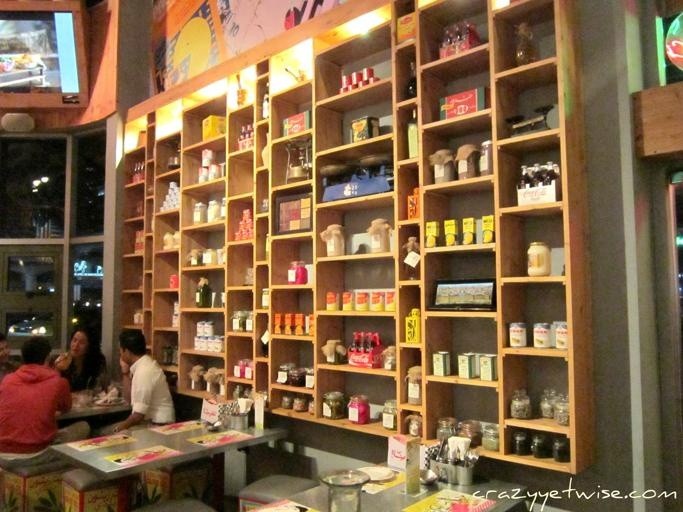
[317,468,371,512]
[456,465,474,487]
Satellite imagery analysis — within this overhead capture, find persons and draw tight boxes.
[0,334,91,472]
[0,332,21,381]
[53,327,106,392]
[91,328,176,438]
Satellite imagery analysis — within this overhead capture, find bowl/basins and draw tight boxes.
[420,469,438,485]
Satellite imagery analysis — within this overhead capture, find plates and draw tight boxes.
[93,397,126,406]
[356,466,395,482]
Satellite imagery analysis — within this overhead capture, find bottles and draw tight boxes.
[132,19,573,465]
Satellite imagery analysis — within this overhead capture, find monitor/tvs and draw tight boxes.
[0,2,89,109]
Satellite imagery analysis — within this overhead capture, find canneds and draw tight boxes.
[326,290,396,311]
[278,340,422,436]
[160,150,269,401]
[338,67,381,95]
[527,241,551,276]
[289,261,307,284]
[509,320,568,349]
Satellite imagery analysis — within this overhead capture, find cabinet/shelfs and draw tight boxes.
[118,1,592,474]
